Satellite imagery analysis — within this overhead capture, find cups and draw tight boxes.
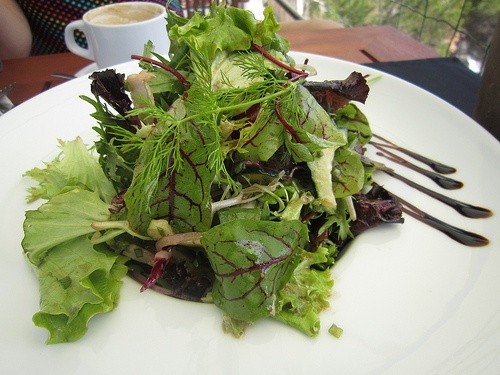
[62,2,171,70]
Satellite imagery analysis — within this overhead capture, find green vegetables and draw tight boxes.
[22,2,404,339]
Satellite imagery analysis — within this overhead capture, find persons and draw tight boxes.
[1,0,185,61]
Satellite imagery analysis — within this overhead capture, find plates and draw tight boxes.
[0,45,499,372]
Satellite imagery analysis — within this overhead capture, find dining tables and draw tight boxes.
[1,22,483,128]
